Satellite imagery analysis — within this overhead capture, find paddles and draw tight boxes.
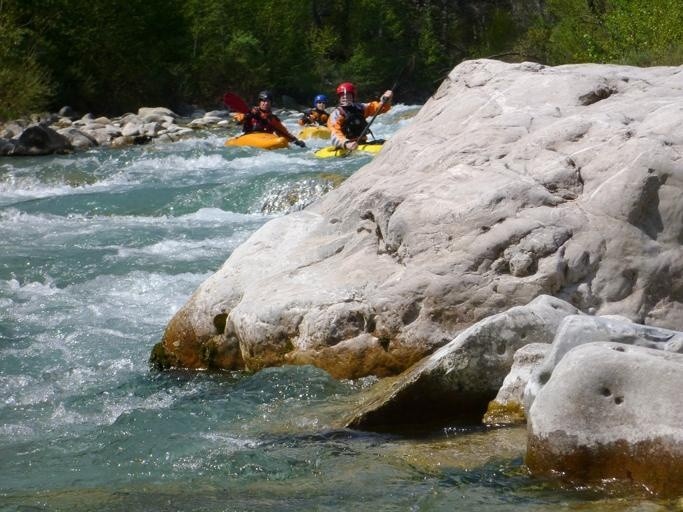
[339,82,397,159]
[282,95,326,127]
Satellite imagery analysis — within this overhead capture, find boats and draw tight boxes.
[316,141,385,158]
[224,133,288,149]
[298,125,331,139]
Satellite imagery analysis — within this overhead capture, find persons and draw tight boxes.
[295,94,332,127]
[326,81,394,151]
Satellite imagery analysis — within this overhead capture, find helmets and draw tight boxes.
[258,90,272,100]
[336,82,357,95]
[313,94,327,105]
